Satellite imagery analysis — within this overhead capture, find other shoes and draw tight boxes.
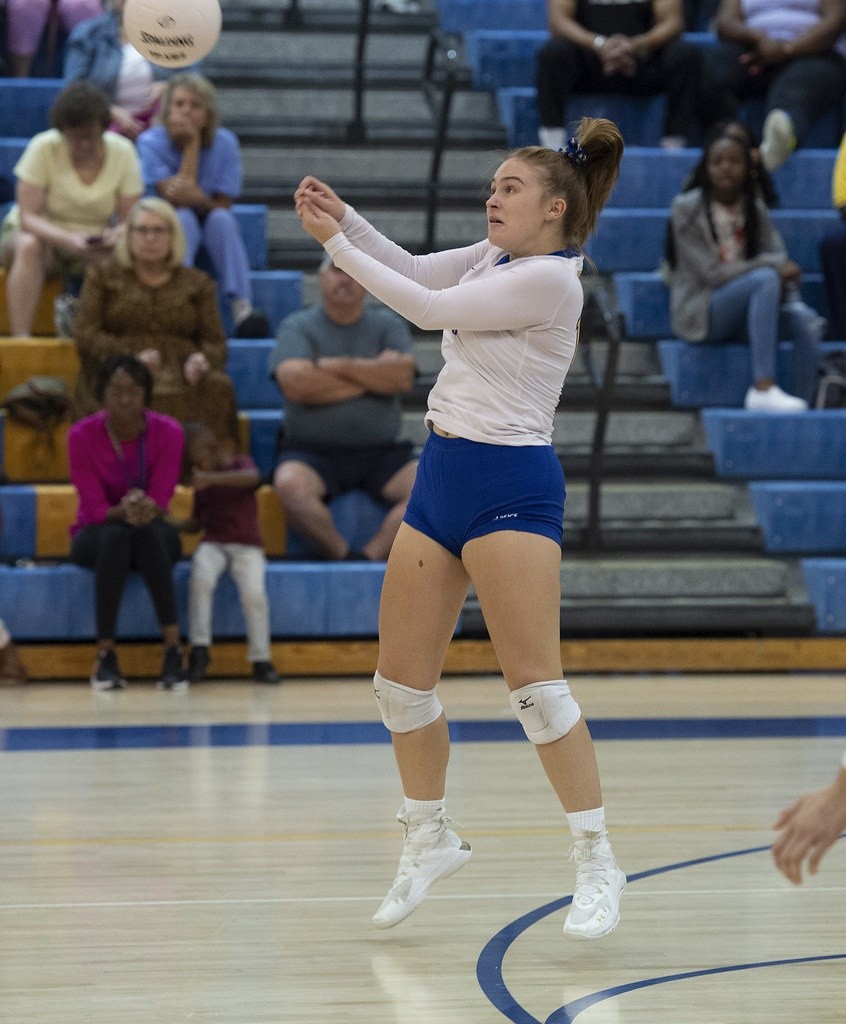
[759,108,795,172]
[190,648,210,679]
[235,310,268,339]
[254,663,278,685]
[0,641,27,687]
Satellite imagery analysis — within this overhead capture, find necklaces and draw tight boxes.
[106,413,146,492]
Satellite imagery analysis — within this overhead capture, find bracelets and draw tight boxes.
[591,35,608,52]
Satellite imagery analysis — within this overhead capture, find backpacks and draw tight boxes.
[6,378,72,465]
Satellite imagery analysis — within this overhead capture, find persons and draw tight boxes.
[771,750,846,885]
[292,118,625,943]
[685,0,845,175]
[72,194,234,484]
[534,0,712,157]
[183,409,284,684]
[271,248,421,564]
[136,72,268,340]
[669,133,820,413]
[0,0,179,140]
[0,83,146,340]
[67,355,191,693]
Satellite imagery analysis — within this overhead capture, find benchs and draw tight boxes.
[436,0,846,635]
[0,64,462,638]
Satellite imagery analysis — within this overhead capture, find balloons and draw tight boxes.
[121,0,223,69]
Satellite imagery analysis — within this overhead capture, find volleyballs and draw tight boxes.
[122,1,223,70]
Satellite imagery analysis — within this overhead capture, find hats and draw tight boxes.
[319,247,332,273]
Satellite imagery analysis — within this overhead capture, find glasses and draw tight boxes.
[134,225,169,237]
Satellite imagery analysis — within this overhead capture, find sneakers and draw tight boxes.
[563,823,627,942]
[370,805,472,930]
[158,649,189,690]
[90,652,127,691]
[744,383,808,412]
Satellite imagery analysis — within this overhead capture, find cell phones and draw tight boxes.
[86,237,102,244]
